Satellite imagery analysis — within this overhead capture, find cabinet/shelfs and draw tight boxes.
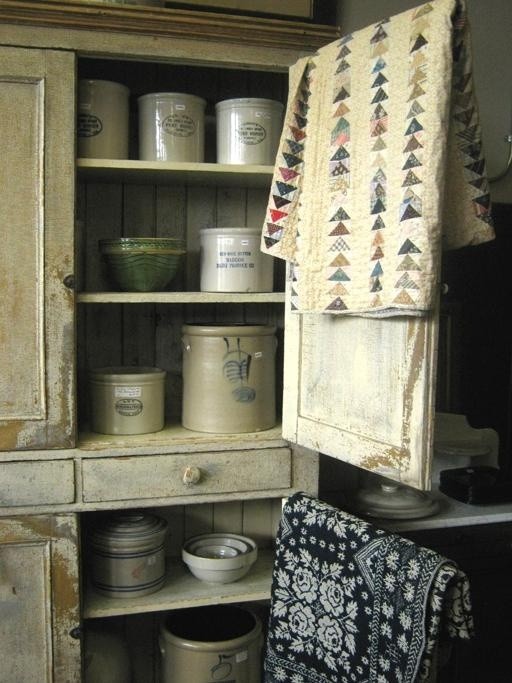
[0,451,325,683]
[2,0,467,453]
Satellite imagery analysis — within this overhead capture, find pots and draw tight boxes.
[86,515,171,598]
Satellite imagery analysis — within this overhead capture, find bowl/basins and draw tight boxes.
[194,545,240,560]
[97,236,187,293]
[186,538,251,559]
[181,532,259,584]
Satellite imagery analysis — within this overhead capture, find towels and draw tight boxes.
[282,316,439,493]
[259,0,497,315]
[264,492,475,683]
